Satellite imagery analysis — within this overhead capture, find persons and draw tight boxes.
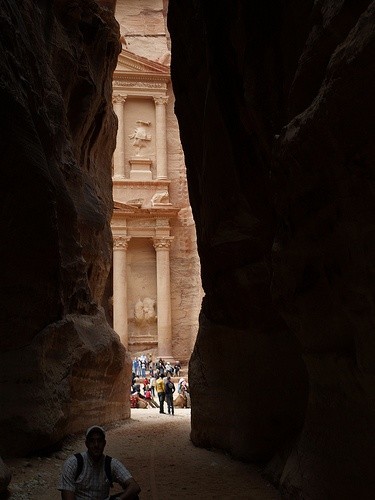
[130,353,190,416]
[57,426,142,500]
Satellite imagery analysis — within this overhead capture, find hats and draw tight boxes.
[85,425,107,441]
